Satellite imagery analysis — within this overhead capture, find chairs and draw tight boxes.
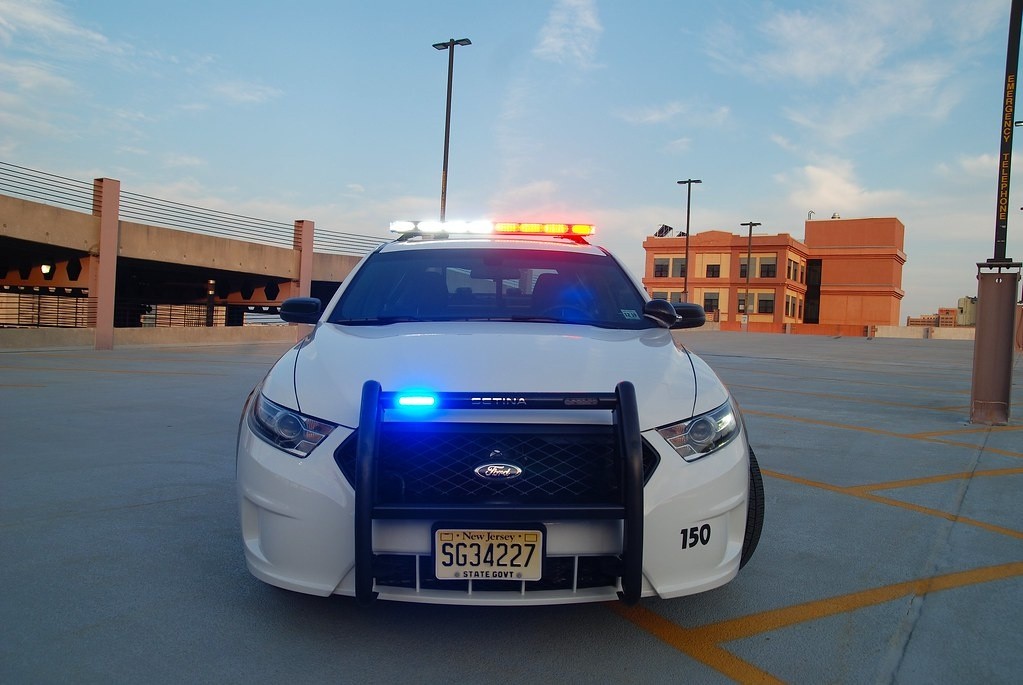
[531,273,563,311]
[409,272,448,312]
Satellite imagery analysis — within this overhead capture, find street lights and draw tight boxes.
[739,222,762,315]
[431,37,472,223]
[676,178,703,303]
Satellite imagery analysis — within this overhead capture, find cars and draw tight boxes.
[235,218,767,610]
